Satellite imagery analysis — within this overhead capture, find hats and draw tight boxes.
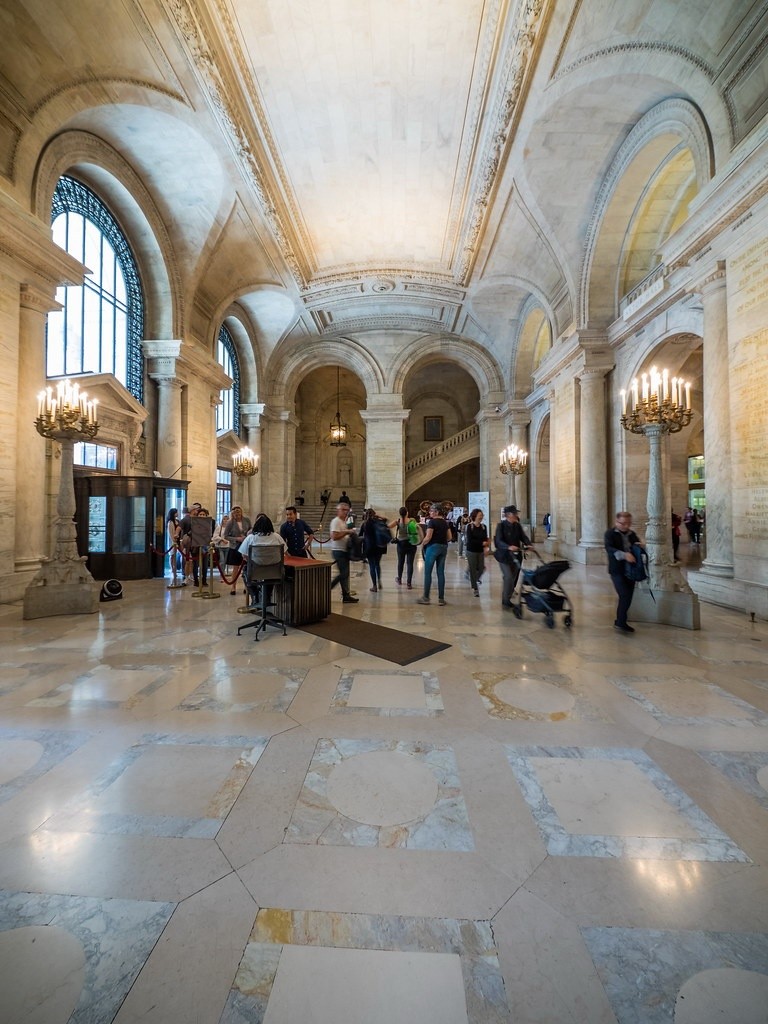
[504,505,520,513]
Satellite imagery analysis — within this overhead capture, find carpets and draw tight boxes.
[252,611,452,667]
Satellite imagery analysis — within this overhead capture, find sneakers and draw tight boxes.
[415,596,429,604]
[343,597,359,603]
[439,600,445,606]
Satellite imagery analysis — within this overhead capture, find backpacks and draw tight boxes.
[625,544,650,582]
[407,517,422,545]
[374,518,391,547]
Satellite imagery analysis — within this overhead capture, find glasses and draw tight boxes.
[618,521,632,526]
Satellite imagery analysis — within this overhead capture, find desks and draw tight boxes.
[259,555,333,626]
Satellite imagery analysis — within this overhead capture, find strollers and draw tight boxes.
[506,546,575,628]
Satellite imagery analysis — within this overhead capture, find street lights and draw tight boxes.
[23,379,101,620]
[498,444,528,507]
[231,446,259,518]
[620,365,701,629]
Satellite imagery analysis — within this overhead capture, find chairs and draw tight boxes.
[238,544,293,641]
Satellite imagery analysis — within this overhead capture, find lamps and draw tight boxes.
[232,445,259,477]
[33,378,101,442]
[619,365,695,436]
[330,366,349,447]
[498,443,529,477]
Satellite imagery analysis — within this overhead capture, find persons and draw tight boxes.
[166,508,179,573]
[359,508,387,592]
[417,504,452,605]
[454,508,470,559]
[295,490,305,506]
[671,507,681,560]
[387,507,418,589]
[175,503,215,587]
[467,509,489,598]
[238,514,287,604]
[281,507,313,557]
[321,490,328,505]
[604,512,646,633]
[684,506,705,544]
[548,516,550,537]
[224,506,251,594]
[329,503,359,603]
[213,515,229,582]
[339,491,351,507]
[495,505,533,609]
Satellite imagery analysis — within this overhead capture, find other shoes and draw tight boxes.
[396,577,401,584]
[614,620,634,633]
[407,583,412,589]
[473,589,479,597]
[503,600,515,609]
[230,590,235,595]
[203,582,208,586]
[370,586,377,592]
[244,590,246,593]
[194,582,198,587]
